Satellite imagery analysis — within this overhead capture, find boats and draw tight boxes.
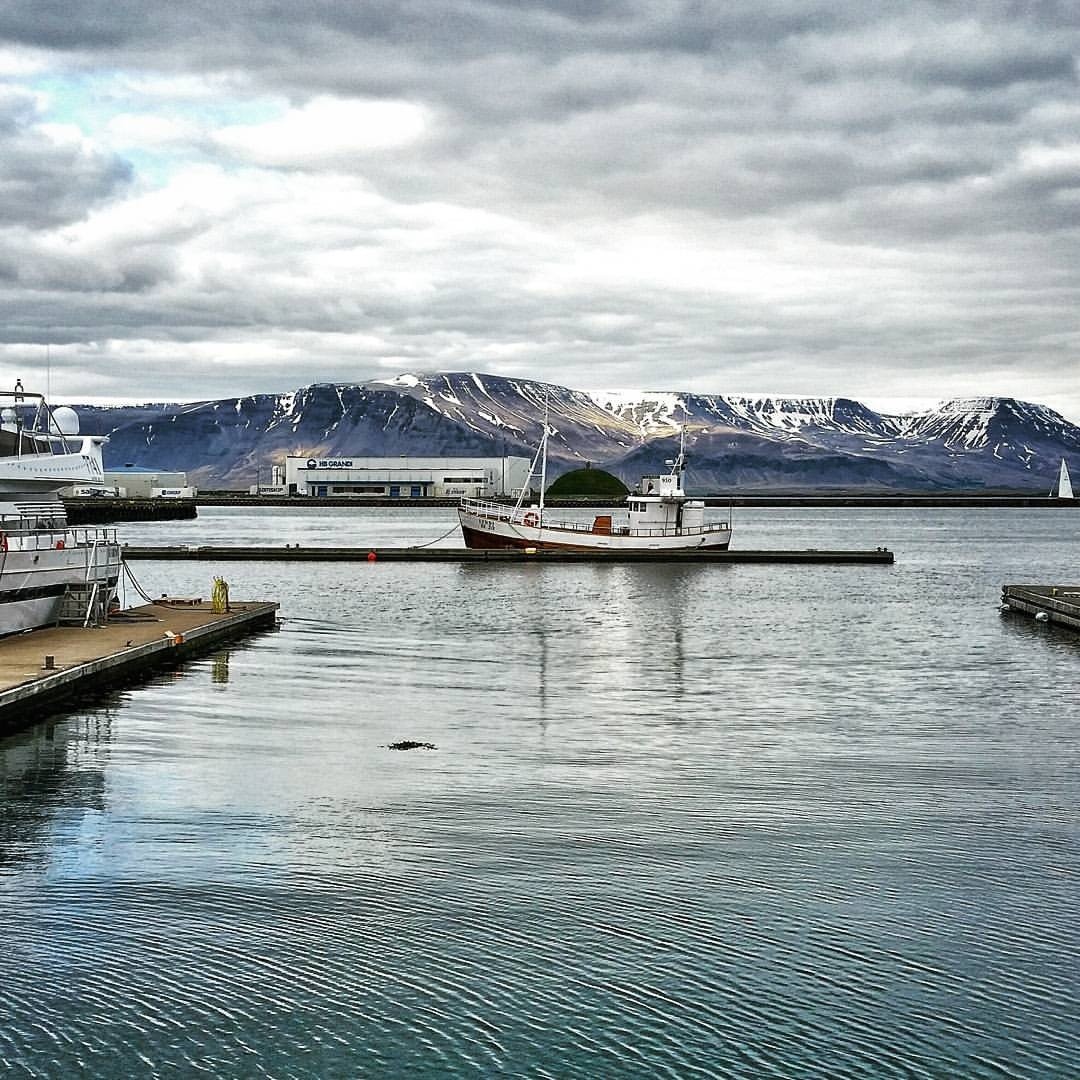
[457,382,733,551]
[0,378,110,519]
[0,528,125,638]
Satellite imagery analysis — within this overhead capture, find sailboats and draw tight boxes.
[1049,458,1075,499]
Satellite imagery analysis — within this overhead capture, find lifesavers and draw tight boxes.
[524,512,538,527]
